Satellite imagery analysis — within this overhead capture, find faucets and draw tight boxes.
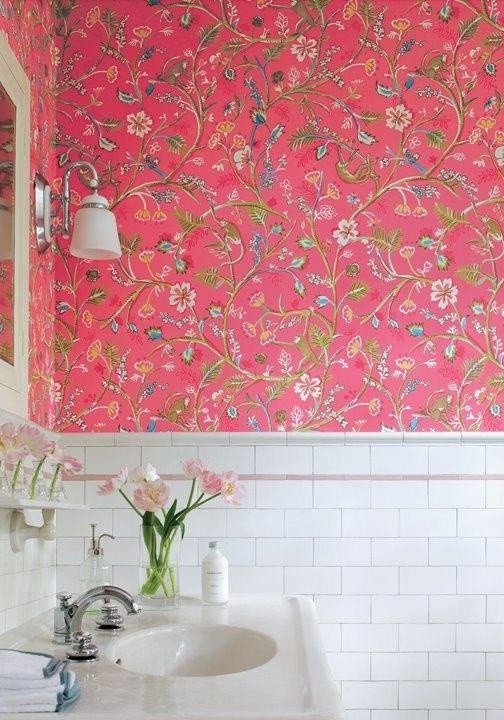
[54,585,141,644]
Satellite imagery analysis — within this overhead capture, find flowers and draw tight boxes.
[96,458,246,597]
[0,422,84,501]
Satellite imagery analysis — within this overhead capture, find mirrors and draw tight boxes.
[0,31,30,420]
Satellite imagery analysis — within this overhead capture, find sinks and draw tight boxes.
[102,623,279,679]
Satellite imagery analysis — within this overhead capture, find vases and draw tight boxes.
[138,526,179,611]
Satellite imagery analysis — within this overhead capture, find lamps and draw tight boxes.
[35,161,122,261]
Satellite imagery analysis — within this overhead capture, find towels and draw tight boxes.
[0,648,82,713]
